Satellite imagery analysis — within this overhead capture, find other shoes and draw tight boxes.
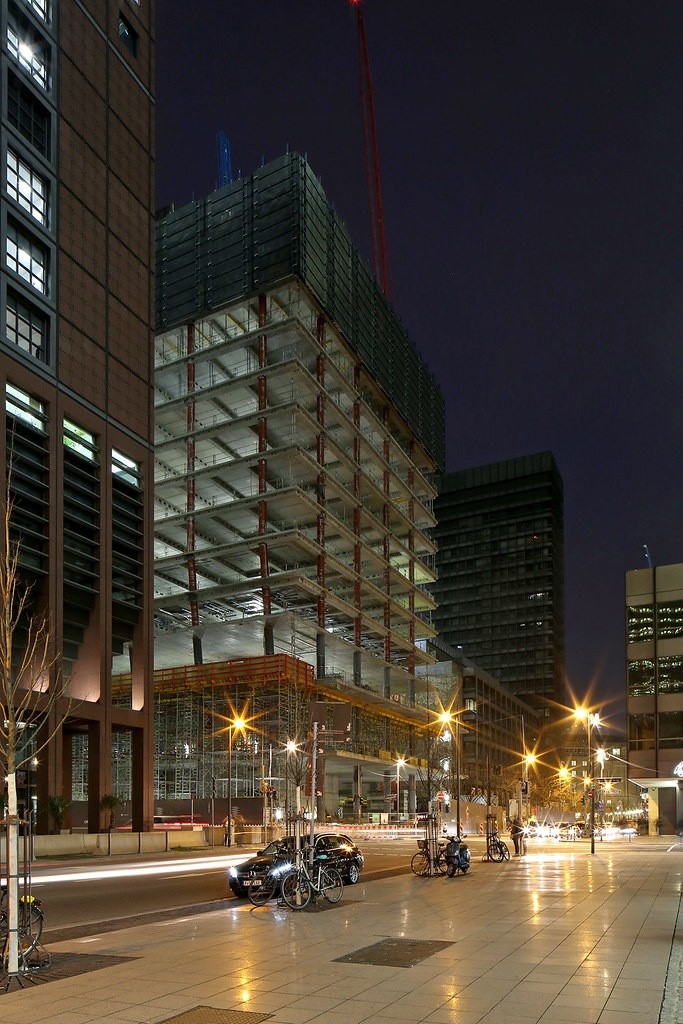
[225,845,226,846]
[512,853,519,857]
[521,852,527,856]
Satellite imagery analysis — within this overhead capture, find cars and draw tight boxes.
[229,833,364,897]
[523,820,614,839]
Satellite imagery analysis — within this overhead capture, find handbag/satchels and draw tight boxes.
[510,834,513,839]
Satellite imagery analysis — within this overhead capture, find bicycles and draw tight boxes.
[248,844,343,907]
[1,876,44,966]
[486,832,510,863]
[411,838,448,876]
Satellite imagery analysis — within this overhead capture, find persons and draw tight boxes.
[510,818,524,857]
[222,814,235,846]
[236,815,246,845]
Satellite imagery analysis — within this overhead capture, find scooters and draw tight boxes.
[442,835,471,877]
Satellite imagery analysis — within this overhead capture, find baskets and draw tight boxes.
[417,840,426,849]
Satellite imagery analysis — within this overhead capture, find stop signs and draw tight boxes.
[438,793,444,799]
[589,792,592,797]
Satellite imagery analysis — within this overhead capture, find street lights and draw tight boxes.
[228,718,246,847]
[575,708,590,773]
[397,758,405,822]
[284,741,295,837]
